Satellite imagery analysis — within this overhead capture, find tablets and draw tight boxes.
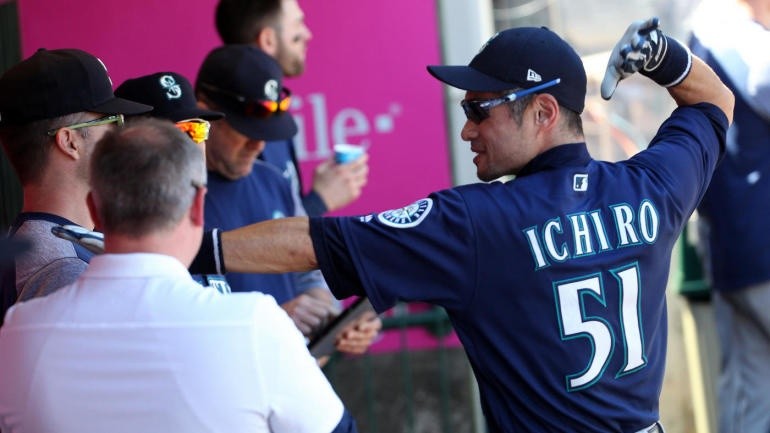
[306,295,374,358]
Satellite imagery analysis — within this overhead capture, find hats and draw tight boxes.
[112,72,227,125]
[427,27,586,114]
[195,44,298,140]
[0,47,152,131]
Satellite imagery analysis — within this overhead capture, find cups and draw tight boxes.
[332,144,364,164]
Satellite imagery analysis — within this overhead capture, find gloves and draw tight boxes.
[600,17,692,100]
[49,224,223,277]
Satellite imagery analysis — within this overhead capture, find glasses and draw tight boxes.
[47,113,125,134]
[175,123,210,144]
[460,78,560,124]
[204,85,292,117]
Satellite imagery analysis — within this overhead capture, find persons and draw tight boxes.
[51,17,736,433]
[0,47,153,330]
[215,0,369,218]
[192,45,382,368]
[92,69,231,295]
[687,0,770,433]
[0,118,362,433]
